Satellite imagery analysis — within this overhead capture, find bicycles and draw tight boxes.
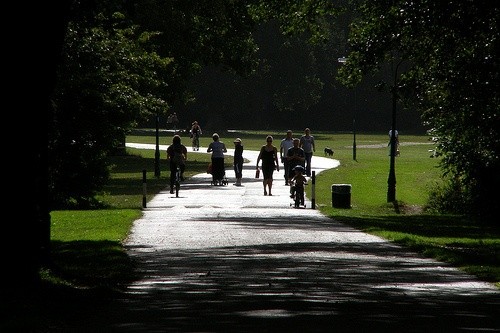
[171,158,187,198]
[289,183,309,208]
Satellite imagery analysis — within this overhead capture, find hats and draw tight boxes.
[292,166,303,171]
[234,138,242,142]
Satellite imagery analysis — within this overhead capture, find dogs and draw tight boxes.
[324,147,334,156]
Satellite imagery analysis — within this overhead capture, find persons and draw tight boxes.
[233,138,244,186]
[290,165,305,205]
[298,126,315,177]
[256,135,280,196]
[279,130,298,185]
[387,129,401,156]
[287,139,306,186]
[191,121,202,149]
[207,133,227,186]
[166,135,187,194]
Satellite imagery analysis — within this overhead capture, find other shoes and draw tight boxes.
[264,192,272,196]
[233,181,242,186]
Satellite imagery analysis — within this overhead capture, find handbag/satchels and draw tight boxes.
[255,171,259,179]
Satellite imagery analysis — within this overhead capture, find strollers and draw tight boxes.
[210,164,229,186]
